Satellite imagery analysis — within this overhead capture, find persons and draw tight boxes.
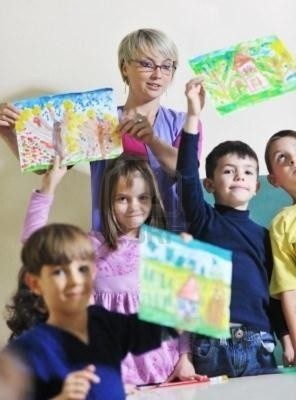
[0,221,195,400]
[180,76,295,377]
[1,28,202,241]
[17,153,197,386]
[265,129,296,362]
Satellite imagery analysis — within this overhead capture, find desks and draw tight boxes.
[127,367,296,399]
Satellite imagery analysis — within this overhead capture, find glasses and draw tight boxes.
[132,59,176,75]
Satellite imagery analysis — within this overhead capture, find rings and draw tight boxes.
[138,118,143,123]
